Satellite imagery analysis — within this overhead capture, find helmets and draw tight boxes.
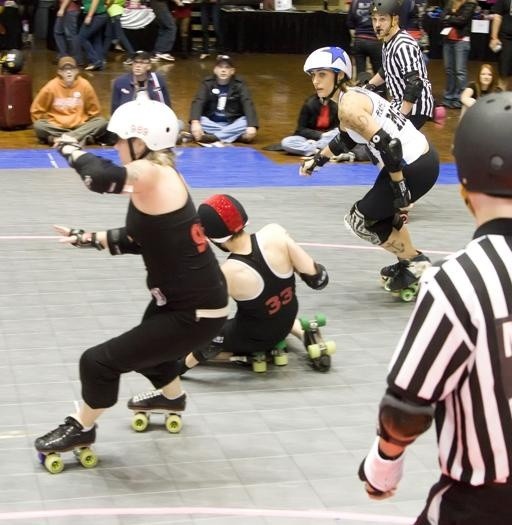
[197,193,248,238]
[0,49,26,72]
[107,100,179,151]
[369,0,400,16]
[303,45,353,78]
[452,89,512,195]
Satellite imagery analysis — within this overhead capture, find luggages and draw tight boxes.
[0,73,34,129]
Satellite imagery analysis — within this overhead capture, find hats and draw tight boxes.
[57,56,76,69]
[215,55,233,66]
[131,50,150,59]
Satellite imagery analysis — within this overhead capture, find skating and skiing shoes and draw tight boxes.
[380,250,424,291]
[400,210,409,224]
[34,415,99,473]
[299,313,337,370]
[229,341,288,373]
[128,390,188,432]
[385,254,432,301]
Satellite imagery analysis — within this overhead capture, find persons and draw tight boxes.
[480,0,512,92]
[31,57,108,147]
[151,0,177,61]
[201,0,225,59]
[34,101,228,473]
[52,0,81,66]
[282,92,370,162]
[112,50,172,121]
[361,0,435,129]
[300,46,439,302]
[189,55,259,143]
[459,63,503,123]
[78,0,109,70]
[358,84,512,525]
[101,0,139,65]
[171,0,193,57]
[198,194,331,375]
[438,0,476,108]
[347,0,382,82]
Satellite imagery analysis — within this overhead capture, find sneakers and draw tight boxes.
[84,64,103,70]
[441,98,452,108]
[155,52,175,61]
[453,100,462,107]
[123,58,133,65]
[181,131,193,143]
[150,56,161,62]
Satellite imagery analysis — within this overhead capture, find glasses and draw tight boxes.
[133,60,149,64]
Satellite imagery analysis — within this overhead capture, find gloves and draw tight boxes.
[51,136,82,157]
[301,150,331,176]
[358,435,405,496]
[390,178,414,212]
[70,228,106,251]
